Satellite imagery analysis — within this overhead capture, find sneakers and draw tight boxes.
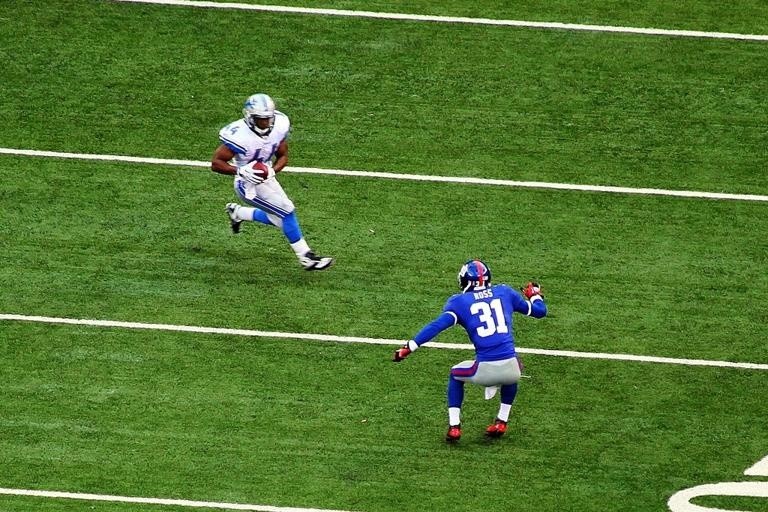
[226,203,244,233]
[446,424,462,442]
[487,416,508,437]
[300,252,333,271]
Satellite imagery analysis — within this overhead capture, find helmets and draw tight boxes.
[458,260,491,291]
[243,93,275,136]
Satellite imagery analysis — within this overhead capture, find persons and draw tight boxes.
[211,93,333,271]
[390,259,547,441]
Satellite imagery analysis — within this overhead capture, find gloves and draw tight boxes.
[236,161,275,185]
[520,282,544,303]
[391,342,411,362]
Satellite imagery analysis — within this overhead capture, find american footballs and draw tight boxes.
[253,162,268,180]
